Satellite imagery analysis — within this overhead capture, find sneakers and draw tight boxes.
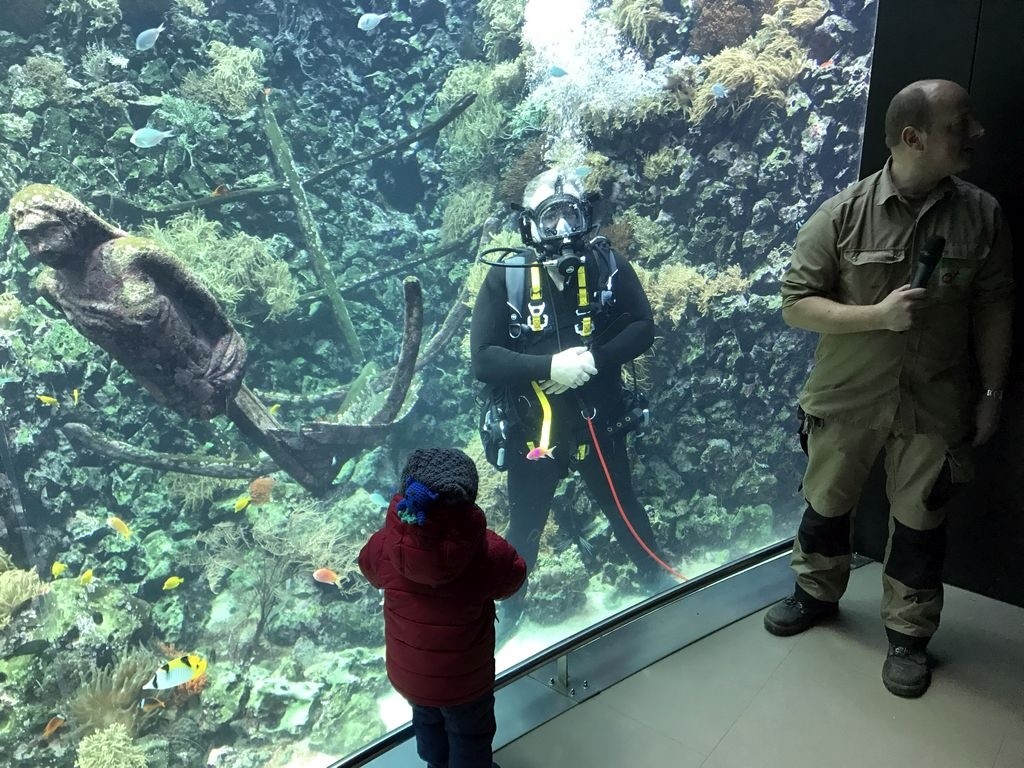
[762,592,841,636]
[881,639,930,697]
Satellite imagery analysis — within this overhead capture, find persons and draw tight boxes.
[470,172,678,649]
[7,184,248,421]
[764,79,1012,698]
[360,449,527,768]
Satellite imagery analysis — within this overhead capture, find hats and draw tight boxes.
[396,445,478,525]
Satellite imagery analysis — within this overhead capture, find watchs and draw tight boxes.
[981,387,1005,402]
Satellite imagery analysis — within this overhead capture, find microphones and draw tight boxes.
[897,235,945,335]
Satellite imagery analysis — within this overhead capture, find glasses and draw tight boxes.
[535,195,590,239]
